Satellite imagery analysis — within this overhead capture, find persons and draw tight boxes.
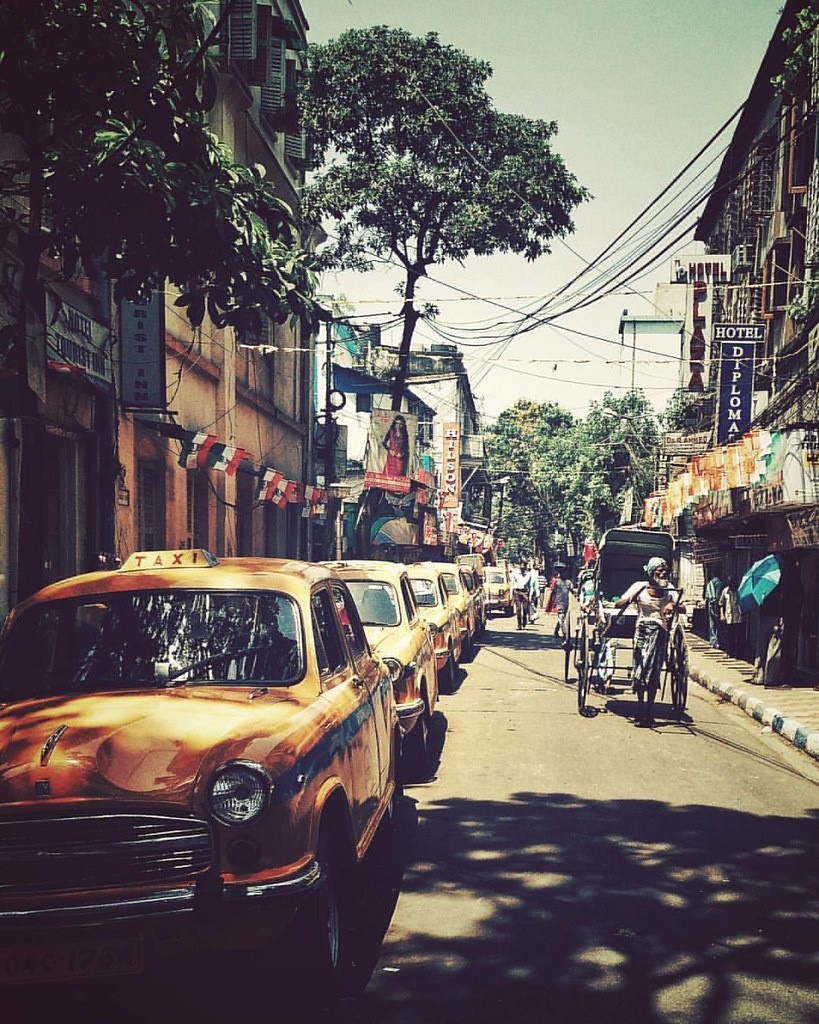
[383,416,409,476]
[581,605,615,695]
[750,573,804,684]
[616,557,686,727]
[705,566,747,659]
[546,566,577,638]
[582,533,597,562]
[512,562,541,631]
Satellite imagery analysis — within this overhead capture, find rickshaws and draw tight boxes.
[563,527,693,726]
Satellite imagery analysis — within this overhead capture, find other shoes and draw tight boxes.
[644,716,657,727]
[554,632,558,637]
[516,625,521,630]
[634,717,645,726]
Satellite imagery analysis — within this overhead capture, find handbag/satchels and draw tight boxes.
[528,602,540,621]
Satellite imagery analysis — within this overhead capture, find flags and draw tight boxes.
[271,479,319,509]
[254,465,285,500]
[445,512,460,533]
[643,430,783,527]
[209,443,245,477]
[177,431,218,469]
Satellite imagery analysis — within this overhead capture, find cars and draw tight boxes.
[1,547,409,989]
[310,554,516,756]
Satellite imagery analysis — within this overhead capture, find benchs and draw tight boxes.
[595,593,639,638]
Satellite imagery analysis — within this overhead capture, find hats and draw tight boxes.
[647,556,667,577]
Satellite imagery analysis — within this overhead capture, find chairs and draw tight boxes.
[359,588,396,623]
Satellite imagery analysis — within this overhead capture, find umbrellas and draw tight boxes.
[736,553,786,613]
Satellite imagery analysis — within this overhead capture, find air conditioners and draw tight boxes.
[731,243,754,274]
